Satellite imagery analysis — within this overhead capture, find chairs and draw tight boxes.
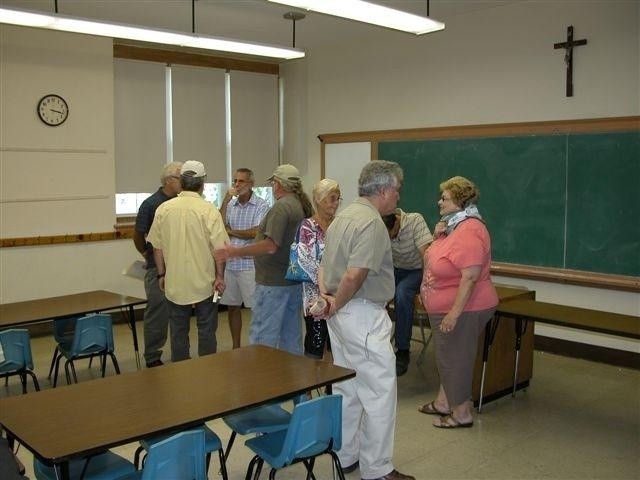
[49,313,120,388]
[0,328,40,393]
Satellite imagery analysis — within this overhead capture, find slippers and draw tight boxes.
[432,414,473,428]
[419,402,451,417]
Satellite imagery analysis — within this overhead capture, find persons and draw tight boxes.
[133,162,183,368]
[382,207,434,377]
[309,159,416,480]
[219,169,270,350]
[213,164,305,355]
[295,179,343,400]
[418,176,500,428]
[145,160,230,362]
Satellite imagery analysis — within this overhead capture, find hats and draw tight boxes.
[267,165,301,184]
[180,161,206,178]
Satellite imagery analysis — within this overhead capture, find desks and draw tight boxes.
[388,283,536,407]
[0,290,149,371]
[0,343,356,479]
[477,298,639,414]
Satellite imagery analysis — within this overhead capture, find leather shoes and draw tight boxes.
[342,459,415,480]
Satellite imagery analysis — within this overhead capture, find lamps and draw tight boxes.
[268,0,446,36]
[0,0,311,66]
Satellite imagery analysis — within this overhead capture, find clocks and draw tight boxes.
[37,94,69,126]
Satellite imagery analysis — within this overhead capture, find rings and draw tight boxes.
[446,328,451,331]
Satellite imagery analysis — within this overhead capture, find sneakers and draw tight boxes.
[146,360,164,367]
[396,354,410,374]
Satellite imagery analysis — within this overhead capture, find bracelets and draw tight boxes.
[157,272,165,280]
[141,250,148,256]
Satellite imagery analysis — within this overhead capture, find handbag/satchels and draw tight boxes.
[285,243,320,282]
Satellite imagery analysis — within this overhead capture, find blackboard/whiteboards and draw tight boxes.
[318,115,640,291]
